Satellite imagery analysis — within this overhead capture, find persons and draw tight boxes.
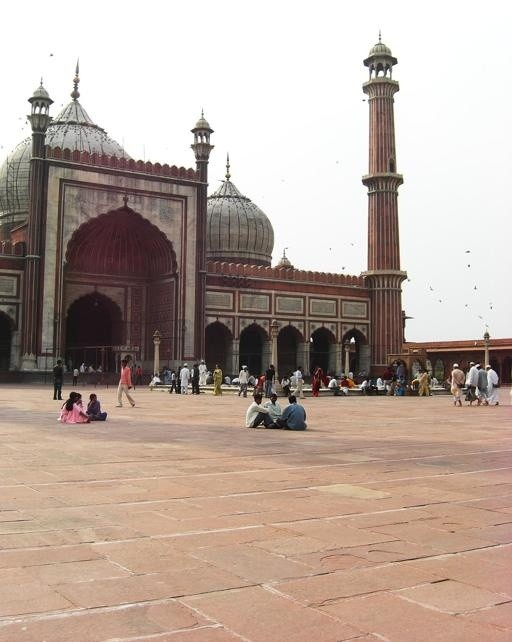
[276,396,306,431]
[444,376,451,391]
[264,395,281,422]
[73,363,103,386]
[281,366,307,399]
[311,367,328,397]
[451,362,498,406]
[58,392,91,425]
[377,369,432,396]
[154,361,231,396]
[117,360,136,406]
[136,366,143,385]
[86,394,108,420]
[245,394,275,430]
[231,364,276,398]
[75,394,84,411]
[54,360,64,399]
[327,375,374,396]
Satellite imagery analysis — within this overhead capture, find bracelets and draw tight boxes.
[86,415,88,417]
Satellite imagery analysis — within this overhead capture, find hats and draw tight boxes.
[201,359,205,363]
[453,363,460,368]
[242,365,249,369]
[469,362,492,368]
[183,363,190,366]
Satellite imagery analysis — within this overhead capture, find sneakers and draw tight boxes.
[116,403,122,407]
[58,398,63,400]
[53,398,57,400]
[130,401,136,407]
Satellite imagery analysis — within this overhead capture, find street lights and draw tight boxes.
[483,330,490,371]
[269,317,279,386]
[343,336,351,377]
[151,330,162,376]
